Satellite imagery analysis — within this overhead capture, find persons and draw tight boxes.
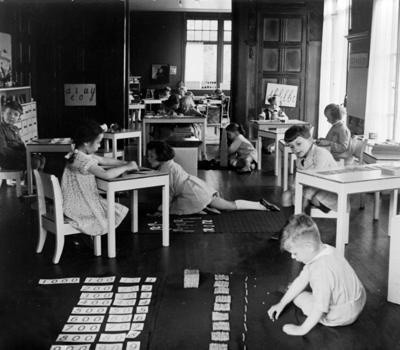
[157,83,226,119]
[225,122,257,174]
[278,125,339,217]
[266,212,367,337]
[145,139,282,217]
[314,102,352,162]
[259,96,289,153]
[59,118,138,246]
[0,100,46,197]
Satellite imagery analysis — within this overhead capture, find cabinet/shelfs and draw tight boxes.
[1,86,38,144]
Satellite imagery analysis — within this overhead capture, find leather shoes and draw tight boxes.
[260,198,280,212]
[202,206,221,215]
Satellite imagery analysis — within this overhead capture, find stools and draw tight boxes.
[0,169,24,199]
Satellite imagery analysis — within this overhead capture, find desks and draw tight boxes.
[293,163,400,260]
[26,127,170,264]
[128,91,227,159]
[250,116,400,218]
